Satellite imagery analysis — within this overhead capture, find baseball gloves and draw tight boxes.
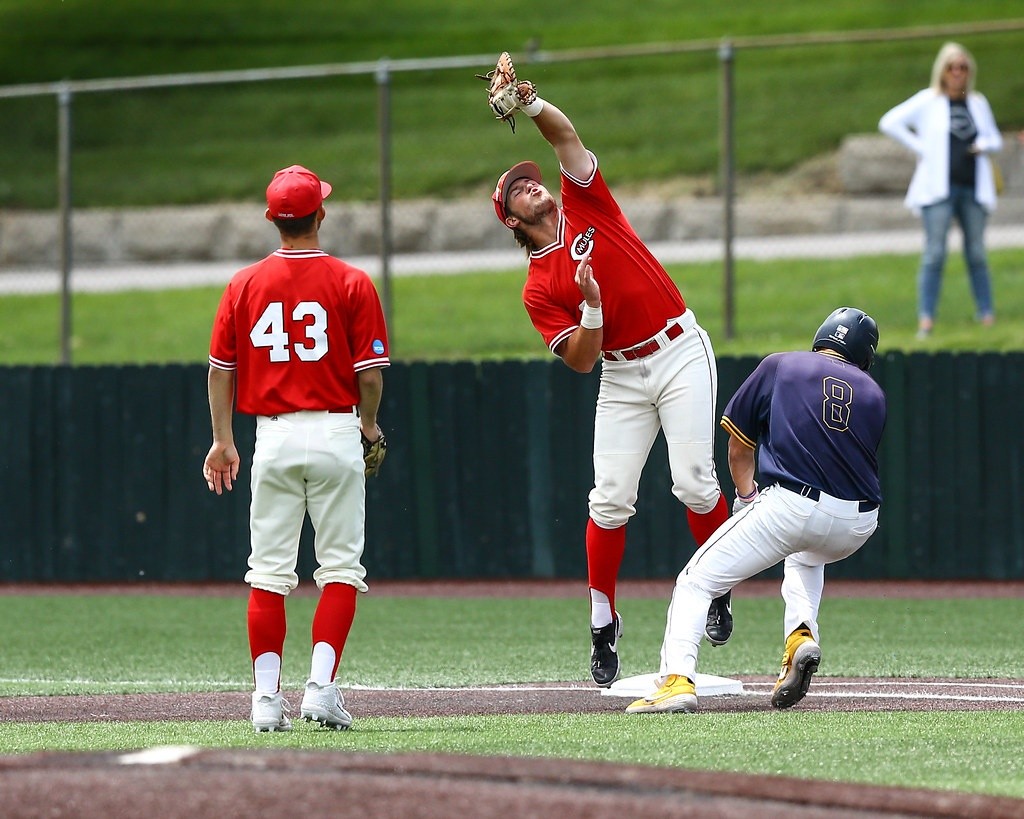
[360,423,387,480]
[472,50,538,135]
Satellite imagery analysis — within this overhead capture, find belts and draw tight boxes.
[781,480,882,512]
[328,406,360,414]
[603,324,683,362]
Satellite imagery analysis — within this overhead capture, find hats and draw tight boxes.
[265,166,332,219]
[492,161,542,224]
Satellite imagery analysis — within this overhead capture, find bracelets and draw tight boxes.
[578,299,604,330]
[519,96,543,118]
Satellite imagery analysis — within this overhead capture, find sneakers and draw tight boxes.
[772,629,821,706]
[625,675,699,712]
[300,679,352,730]
[704,590,734,646]
[591,610,625,688]
[252,689,292,732]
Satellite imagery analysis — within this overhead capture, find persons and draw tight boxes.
[203,164,392,734]
[627,306,886,712]
[879,40,1008,335]
[492,52,732,687]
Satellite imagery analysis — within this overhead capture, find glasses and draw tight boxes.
[945,64,968,73]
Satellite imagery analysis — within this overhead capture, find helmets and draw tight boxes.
[813,305,878,369]
